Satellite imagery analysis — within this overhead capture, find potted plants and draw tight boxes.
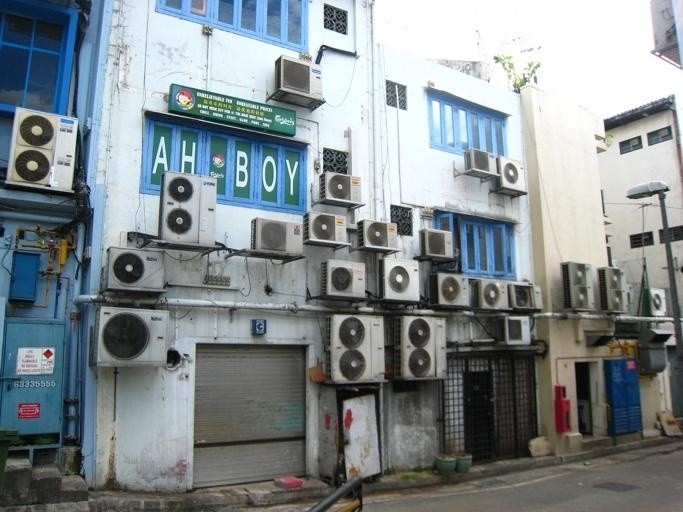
[436,452,472,476]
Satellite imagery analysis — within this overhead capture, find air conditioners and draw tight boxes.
[598,268,624,311]
[304,211,470,384]
[275,56,325,108]
[643,286,666,316]
[94,246,169,368]
[319,172,362,207]
[561,261,589,309]
[478,277,545,344]
[251,217,303,256]
[158,173,216,252]
[4,107,83,197]
[466,149,526,198]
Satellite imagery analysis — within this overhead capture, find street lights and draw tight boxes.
[626,180,683,368]
